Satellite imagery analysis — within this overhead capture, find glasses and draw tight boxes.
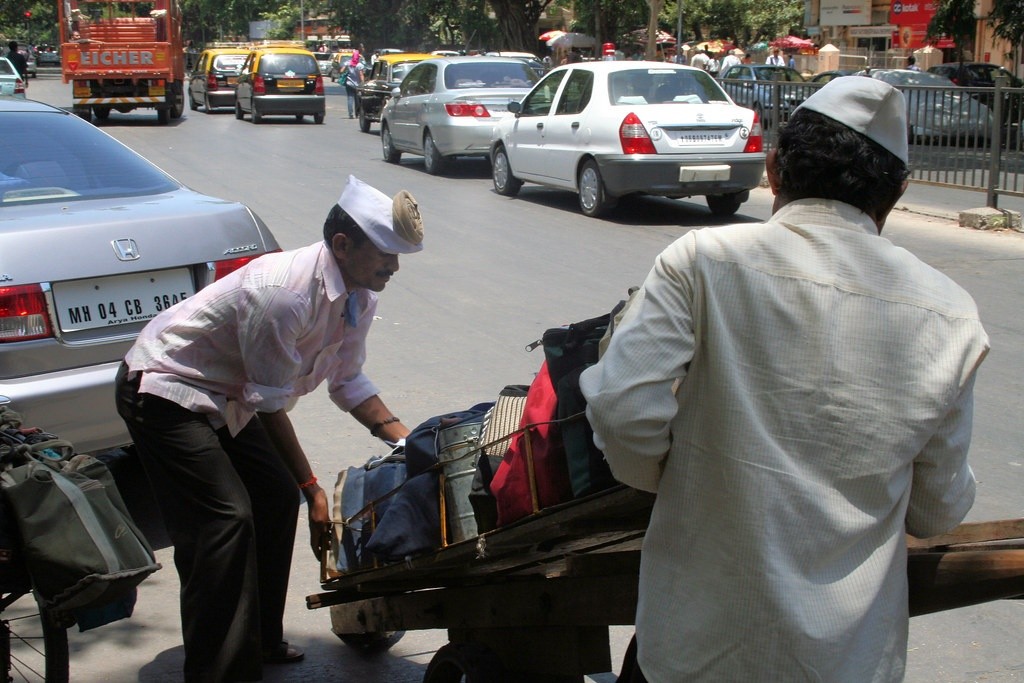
[773,50,779,53]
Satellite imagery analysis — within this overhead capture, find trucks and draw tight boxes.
[58,0,194,126]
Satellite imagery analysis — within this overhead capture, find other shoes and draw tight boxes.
[262,639,305,664]
[349,115,353,119]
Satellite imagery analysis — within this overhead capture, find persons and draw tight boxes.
[616,45,686,64]
[317,41,365,54]
[578,76,992,683]
[114,173,424,683]
[690,49,752,78]
[561,47,582,64]
[68,30,102,43]
[904,55,922,72]
[370,51,380,65]
[765,47,794,80]
[6,41,28,88]
[340,51,364,119]
[543,51,552,68]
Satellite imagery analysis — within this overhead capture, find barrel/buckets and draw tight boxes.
[433,422,495,544]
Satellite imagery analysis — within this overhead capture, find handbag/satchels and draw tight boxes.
[2,441,161,629]
[338,70,349,85]
[330,285,642,567]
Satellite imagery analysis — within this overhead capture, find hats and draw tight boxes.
[338,173,425,256]
[791,75,910,162]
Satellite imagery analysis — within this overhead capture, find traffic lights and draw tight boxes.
[24,9,31,23]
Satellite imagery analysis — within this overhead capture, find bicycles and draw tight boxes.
[0,395,70,683]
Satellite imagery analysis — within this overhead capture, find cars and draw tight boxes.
[489,42,767,218]
[188,40,262,113]
[473,51,549,78]
[809,69,860,86]
[847,69,994,148]
[926,62,1024,127]
[360,53,445,132]
[235,41,326,125]
[2,42,38,79]
[36,45,60,67]
[718,63,812,129]
[375,48,405,57]
[380,56,550,175]
[0,57,26,100]
[430,50,460,58]
[313,49,366,82]
[0,90,285,464]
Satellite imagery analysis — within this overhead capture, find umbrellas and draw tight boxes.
[538,30,564,59]
[692,39,743,56]
[546,31,596,52]
[616,27,676,45]
[768,35,813,50]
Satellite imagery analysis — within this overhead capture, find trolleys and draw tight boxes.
[304,481,1024,683]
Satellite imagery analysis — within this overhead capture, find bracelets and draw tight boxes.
[371,416,400,437]
[297,478,316,489]
